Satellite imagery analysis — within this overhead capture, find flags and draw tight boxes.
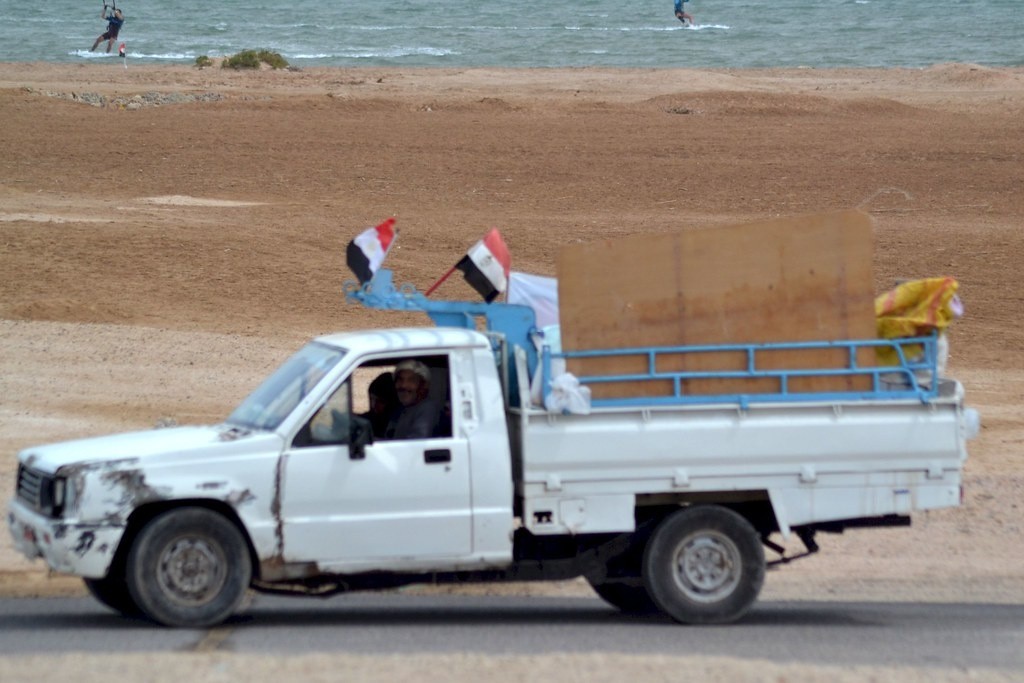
[453,225,514,304]
[345,217,398,291]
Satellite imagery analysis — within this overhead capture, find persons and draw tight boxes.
[346,360,441,442]
[673,0,696,29]
[89,4,125,52]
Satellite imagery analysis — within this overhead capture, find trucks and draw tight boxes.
[6,265,981,634]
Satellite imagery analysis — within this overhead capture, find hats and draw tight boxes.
[394,359,431,381]
